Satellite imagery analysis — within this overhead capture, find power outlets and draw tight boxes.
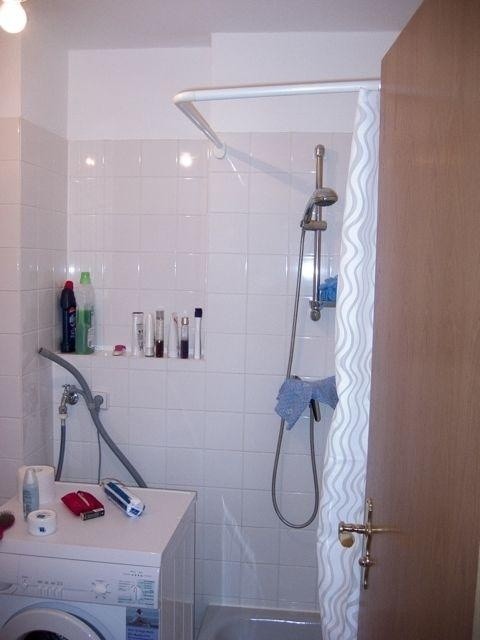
[91,391,107,410]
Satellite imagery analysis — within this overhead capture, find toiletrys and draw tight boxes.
[156,311,165,358]
[132,311,144,355]
[144,312,156,357]
[180,317,189,359]
[22,466,41,523]
[145,312,155,357]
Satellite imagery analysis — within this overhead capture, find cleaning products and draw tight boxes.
[58,281,76,352]
[76,271,96,355]
[22,469,39,520]
[194,307,202,358]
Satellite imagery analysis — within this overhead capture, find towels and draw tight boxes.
[273,376,339,430]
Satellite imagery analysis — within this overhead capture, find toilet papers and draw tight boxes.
[18,465,56,505]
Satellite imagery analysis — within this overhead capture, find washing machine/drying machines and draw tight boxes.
[0,482,196,640]
